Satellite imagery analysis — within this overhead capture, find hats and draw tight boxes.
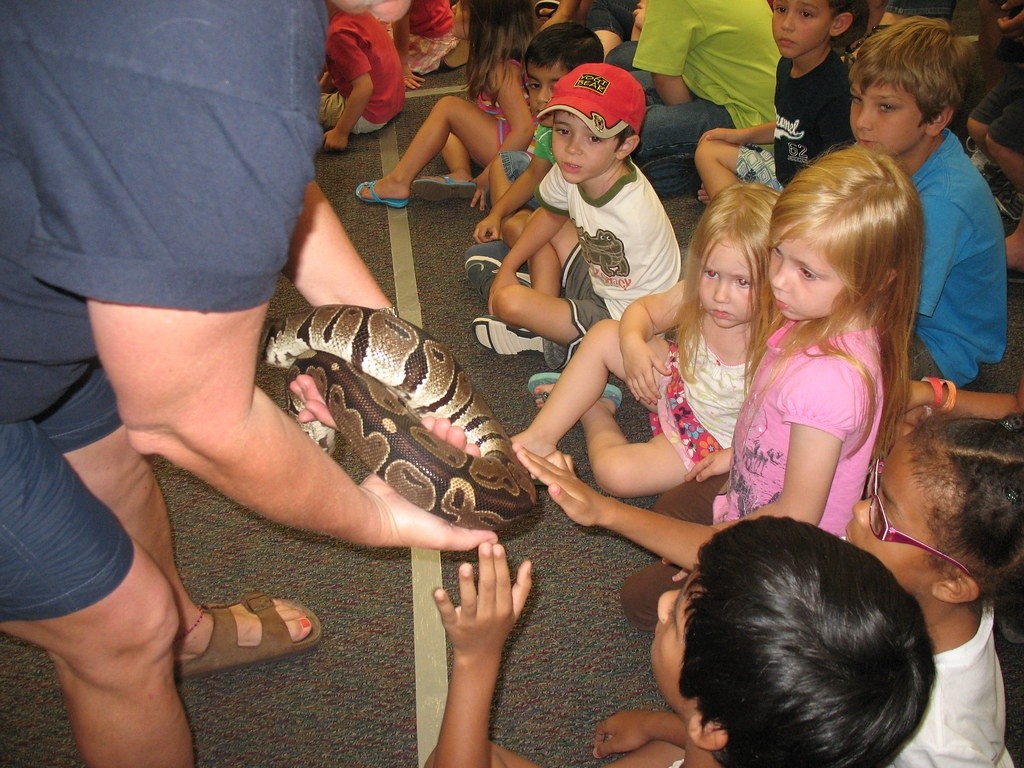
[536,63,645,138]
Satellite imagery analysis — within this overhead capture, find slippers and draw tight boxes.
[535,0,561,20]
[356,182,409,207]
[178,594,321,677]
[530,374,622,415]
[413,176,477,201]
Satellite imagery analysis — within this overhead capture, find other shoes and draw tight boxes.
[442,41,472,67]
[966,138,988,174]
[982,162,1016,195]
[994,190,1024,220]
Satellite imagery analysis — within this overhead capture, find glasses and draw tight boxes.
[867,457,970,576]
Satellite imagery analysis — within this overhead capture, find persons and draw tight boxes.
[0,0,500,768]
[317,0,1024,767]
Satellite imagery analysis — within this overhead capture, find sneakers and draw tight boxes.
[467,255,533,300]
[473,316,546,356]
